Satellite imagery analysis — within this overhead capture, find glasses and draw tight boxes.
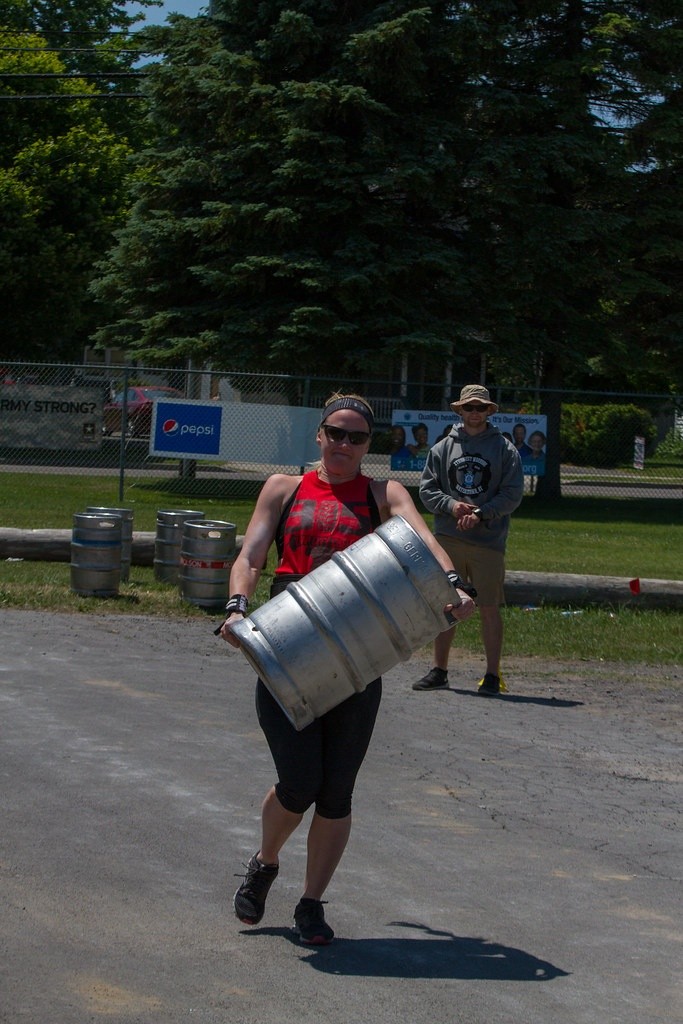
[321,424,370,445]
[461,404,490,412]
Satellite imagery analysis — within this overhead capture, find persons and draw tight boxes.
[218,396,475,946]
[502,432,513,444]
[522,431,545,474]
[411,384,524,695]
[435,425,452,444]
[512,424,532,457]
[407,422,432,471]
[392,425,418,471]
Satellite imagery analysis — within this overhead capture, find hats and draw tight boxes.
[451,384,498,416]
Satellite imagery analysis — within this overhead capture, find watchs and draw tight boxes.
[473,508,482,520]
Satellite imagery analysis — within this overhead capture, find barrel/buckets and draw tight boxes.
[226,514,464,731]
[153,509,205,586]
[86,507,133,583]
[69,512,122,598]
[179,519,237,608]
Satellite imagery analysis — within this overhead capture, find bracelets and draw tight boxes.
[446,570,463,589]
[226,595,248,616]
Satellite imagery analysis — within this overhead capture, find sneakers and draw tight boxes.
[294,898,334,945]
[478,673,500,696]
[233,849,279,924]
[413,667,449,690]
